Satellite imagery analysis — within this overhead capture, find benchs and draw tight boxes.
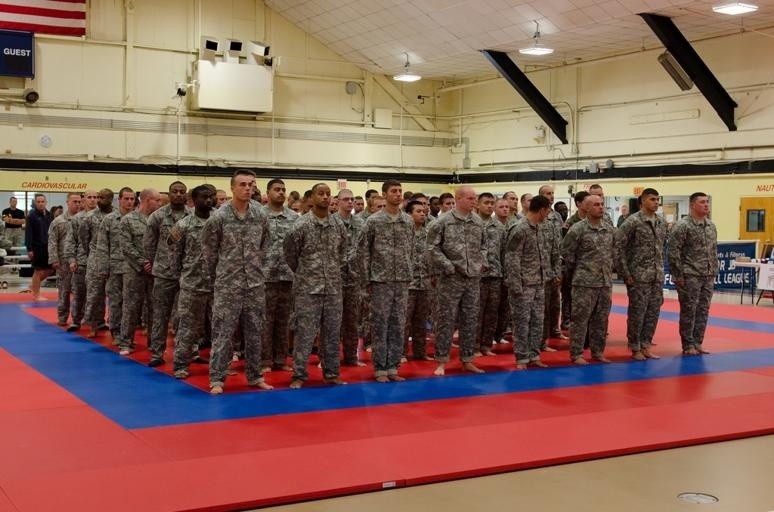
[0,244,35,283]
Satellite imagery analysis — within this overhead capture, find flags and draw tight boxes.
[0,0,86,36]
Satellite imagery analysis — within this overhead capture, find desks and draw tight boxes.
[734,259,774,308]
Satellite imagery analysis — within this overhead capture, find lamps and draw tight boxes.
[710,1,762,18]
[518,19,556,56]
[391,52,422,84]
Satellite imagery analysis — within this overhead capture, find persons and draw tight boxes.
[666,192,719,354]
[617,204,632,280]
[2,197,26,263]
[637,195,656,346]
[613,188,668,361]
